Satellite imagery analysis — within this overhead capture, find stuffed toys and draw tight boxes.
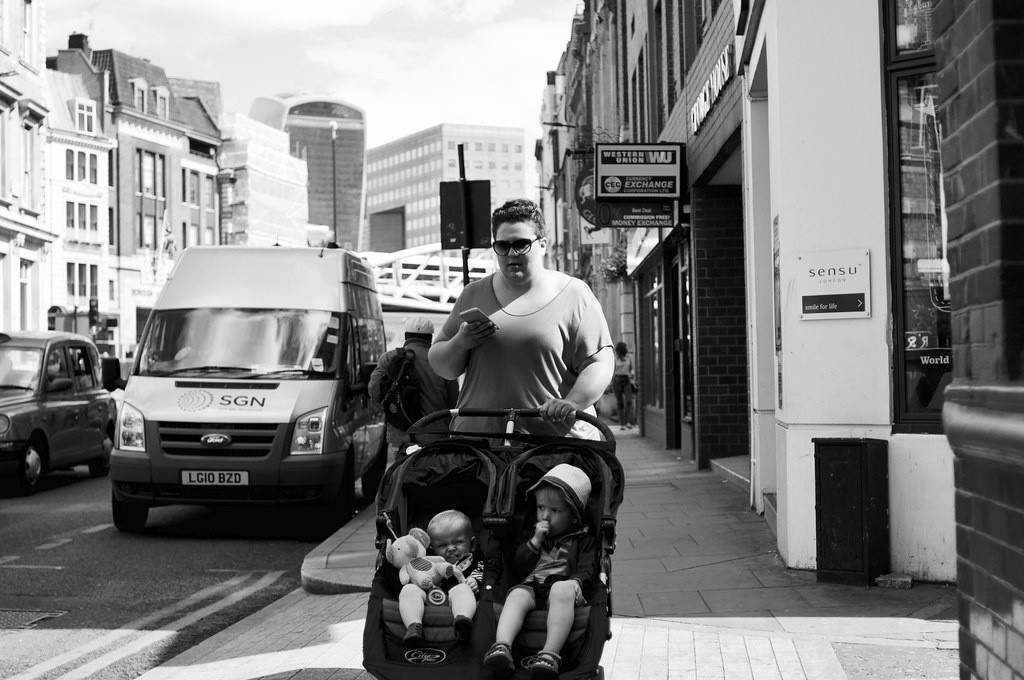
[385,528,454,589]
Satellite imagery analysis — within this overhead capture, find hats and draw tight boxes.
[524,463,592,523]
[404,316,434,333]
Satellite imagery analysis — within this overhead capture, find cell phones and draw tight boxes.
[458,308,499,331]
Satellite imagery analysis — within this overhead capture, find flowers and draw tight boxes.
[598,243,627,285]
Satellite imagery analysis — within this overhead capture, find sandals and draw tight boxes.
[530,651,561,679]
[484,641,514,674]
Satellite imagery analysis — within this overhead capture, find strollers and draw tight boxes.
[363,405,625,678]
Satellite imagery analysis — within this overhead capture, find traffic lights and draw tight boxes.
[89,299,100,334]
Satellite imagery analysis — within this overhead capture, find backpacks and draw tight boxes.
[382,348,424,431]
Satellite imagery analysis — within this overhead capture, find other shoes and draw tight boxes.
[453,614,472,648]
[403,623,423,645]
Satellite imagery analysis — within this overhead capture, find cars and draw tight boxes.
[0,332,117,491]
[105,357,134,410]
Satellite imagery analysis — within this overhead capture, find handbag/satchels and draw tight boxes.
[631,383,638,394]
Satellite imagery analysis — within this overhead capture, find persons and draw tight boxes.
[368,315,460,462]
[485,463,598,676]
[46,352,63,380]
[612,341,636,430]
[427,199,617,448]
[398,510,486,646]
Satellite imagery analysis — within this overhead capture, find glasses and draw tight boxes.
[492,237,540,256]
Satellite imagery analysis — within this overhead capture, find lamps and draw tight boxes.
[543,123,575,128]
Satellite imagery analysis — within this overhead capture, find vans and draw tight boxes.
[102,242,385,530]
